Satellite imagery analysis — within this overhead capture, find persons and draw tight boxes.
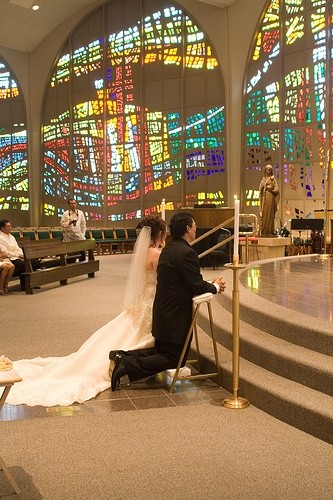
[60,198,87,244]
[0,248,15,295]
[0,219,41,289]
[258,164,280,236]
[97,216,169,355]
[109,211,226,395]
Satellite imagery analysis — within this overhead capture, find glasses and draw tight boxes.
[3,225,11,228]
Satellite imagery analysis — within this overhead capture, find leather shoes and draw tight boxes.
[109,351,126,391]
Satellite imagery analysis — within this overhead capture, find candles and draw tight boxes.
[234,200,240,256]
[162,202,165,222]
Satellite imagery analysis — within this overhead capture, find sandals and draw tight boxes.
[0,284,8,295]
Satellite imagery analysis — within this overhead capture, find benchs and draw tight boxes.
[10,237,99,294]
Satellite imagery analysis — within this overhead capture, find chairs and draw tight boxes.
[11,227,138,254]
[0,369,24,496]
[203,226,231,270]
